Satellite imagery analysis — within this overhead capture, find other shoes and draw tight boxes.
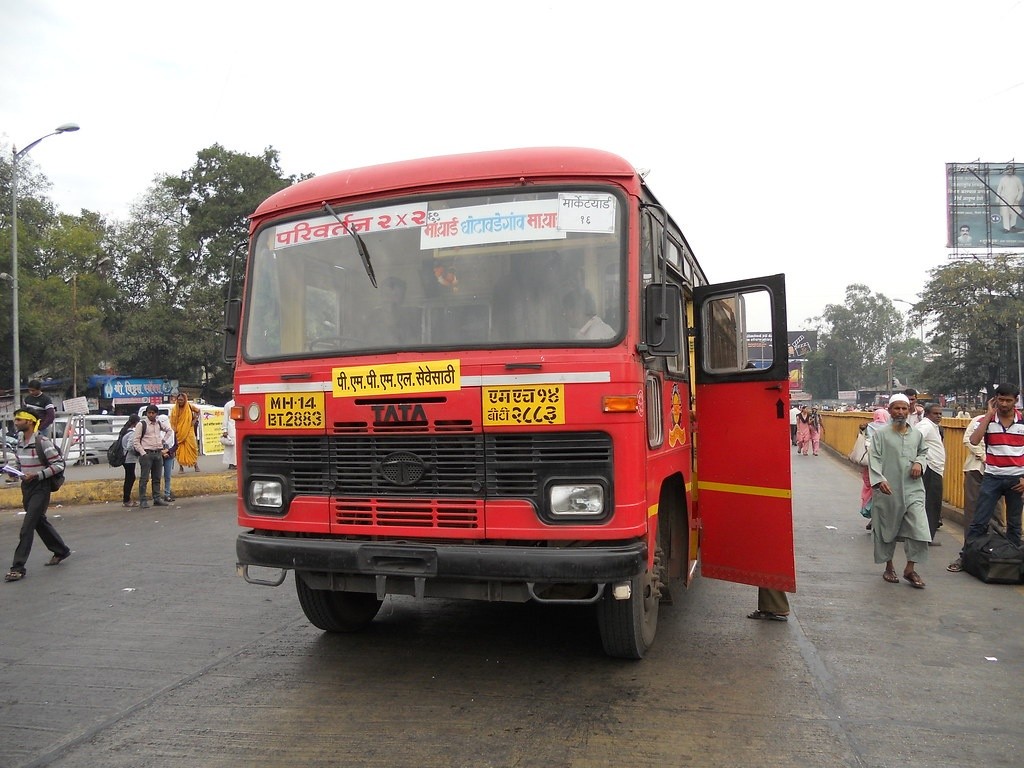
[44,551,70,566]
[179,469,184,474]
[228,465,236,470]
[153,497,168,506]
[139,500,148,509]
[927,540,941,546]
[165,497,175,502]
[195,466,200,472]
[798,447,801,454]
[5,571,22,581]
[866,522,872,530]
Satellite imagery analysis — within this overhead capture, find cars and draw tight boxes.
[0,434,20,474]
[946,395,965,407]
[48,415,131,466]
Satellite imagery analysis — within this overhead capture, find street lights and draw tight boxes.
[829,364,840,398]
[1,124,81,411]
[893,299,923,352]
[65,276,77,397]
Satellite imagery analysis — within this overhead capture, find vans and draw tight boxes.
[138,403,215,442]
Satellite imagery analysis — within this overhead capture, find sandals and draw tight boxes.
[122,500,139,507]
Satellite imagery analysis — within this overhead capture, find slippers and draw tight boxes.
[883,571,899,583]
[947,559,962,572]
[903,571,925,587]
[746,610,788,621]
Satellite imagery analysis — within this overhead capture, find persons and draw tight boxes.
[995,165,1024,232]
[947,383,1024,572]
[221,389,237,469]
[134,405,173,508]
[158,414,179,502]
[962,415,1005,541]
[119,414,140,507]
[5,380,54,482]
[953,406,970,418]
[790,405,801,446]
[747,587,790,621]
[561,287,616,340]
[796,406,825,456]
[958,225,971,242]
[4,407,72,580]
[860,389,945,588]
[356,277,407,340]
[170,393,201,473]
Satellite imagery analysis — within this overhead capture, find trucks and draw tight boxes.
[222,145,796,660]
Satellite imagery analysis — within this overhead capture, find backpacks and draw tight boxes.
[34,435,66,492]
[107,430,135,467]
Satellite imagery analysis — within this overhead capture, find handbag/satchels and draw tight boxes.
[221,435,234,445]
[961,522,1024,586]
[848,424,878,466]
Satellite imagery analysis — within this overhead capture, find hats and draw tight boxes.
[889,393,909,405]
